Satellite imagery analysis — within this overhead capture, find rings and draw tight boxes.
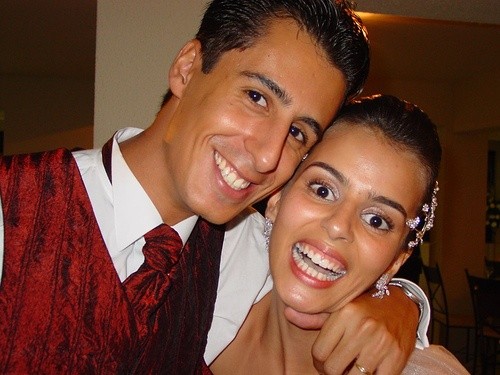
[354,362,372,375]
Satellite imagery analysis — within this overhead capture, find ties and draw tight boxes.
[102,128,183,345]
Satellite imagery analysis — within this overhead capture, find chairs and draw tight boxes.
[419,258,500,371]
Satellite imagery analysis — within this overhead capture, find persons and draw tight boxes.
[0,0,431,375]
[209,93,470,375]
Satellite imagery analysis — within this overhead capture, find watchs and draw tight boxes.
[387,281,424,323]
